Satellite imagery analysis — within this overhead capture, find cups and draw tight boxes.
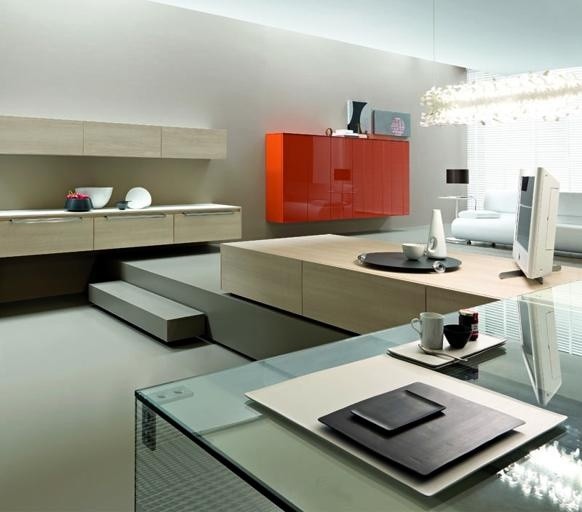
[410,312,472,350]
[402,244,425,261]
[116,201,127,210]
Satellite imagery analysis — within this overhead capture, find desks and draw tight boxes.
[438,195,477,218]
[133,282,582,512]
[220,233,582,404]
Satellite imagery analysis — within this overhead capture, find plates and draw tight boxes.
[125,186,152,209]
[357,251,463,270]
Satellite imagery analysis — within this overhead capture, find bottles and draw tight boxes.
[427,209,447,259]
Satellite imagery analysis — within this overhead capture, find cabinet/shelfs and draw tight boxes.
[265,132,410,223]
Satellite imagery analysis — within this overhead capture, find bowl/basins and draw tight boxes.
[75,187,114,209]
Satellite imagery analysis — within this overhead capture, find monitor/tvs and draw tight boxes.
[500,163,561,283]
[517,296,566,407]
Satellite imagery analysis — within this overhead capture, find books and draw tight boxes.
[333,129,368,139]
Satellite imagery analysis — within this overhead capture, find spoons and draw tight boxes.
[419,343,469,362]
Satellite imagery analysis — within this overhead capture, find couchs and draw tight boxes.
[451,191,516,248]
[553,193,582,258]
